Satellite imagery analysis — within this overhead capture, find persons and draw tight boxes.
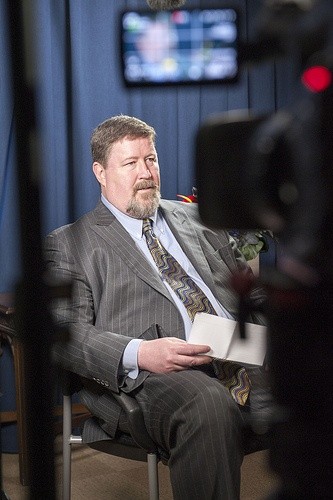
[43,115,290,500]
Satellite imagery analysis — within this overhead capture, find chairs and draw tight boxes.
[62,370,158,500]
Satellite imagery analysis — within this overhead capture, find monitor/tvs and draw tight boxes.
[119,5,241,88]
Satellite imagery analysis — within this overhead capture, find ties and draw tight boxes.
[142,220,252,406]
